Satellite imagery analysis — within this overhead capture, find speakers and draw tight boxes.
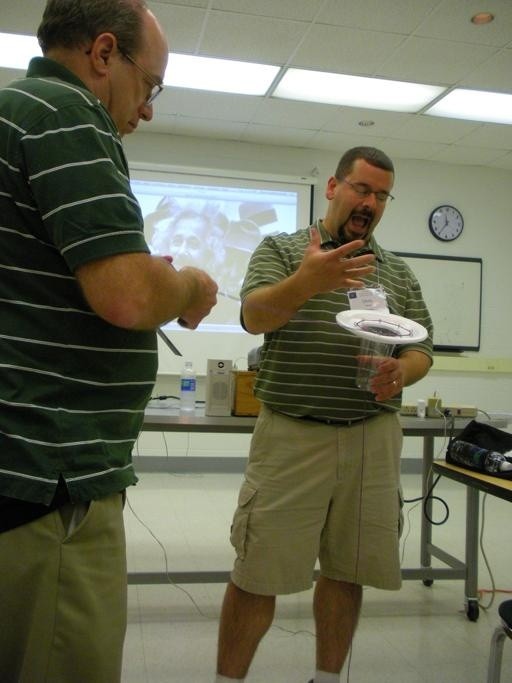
[205,359,233,417]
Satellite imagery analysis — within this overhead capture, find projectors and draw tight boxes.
[247,346,264,371]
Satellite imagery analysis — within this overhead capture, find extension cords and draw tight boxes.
[398,404,479,418]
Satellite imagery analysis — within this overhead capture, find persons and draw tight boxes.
[217,143,438,683]
[147,192,279,285]
[2,1,221,683]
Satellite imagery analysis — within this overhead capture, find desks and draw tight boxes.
[125,403,511,625]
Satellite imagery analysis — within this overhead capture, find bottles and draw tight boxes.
[179,361,196,410]
[451,441,512,473]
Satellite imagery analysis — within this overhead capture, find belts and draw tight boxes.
[277,411,378,427]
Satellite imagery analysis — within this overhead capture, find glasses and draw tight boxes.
[337,177,395,203]
[85,43,164,105]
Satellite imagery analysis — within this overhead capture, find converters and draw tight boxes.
[427,397,443,418]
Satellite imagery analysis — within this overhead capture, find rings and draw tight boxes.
[392,380,398,387]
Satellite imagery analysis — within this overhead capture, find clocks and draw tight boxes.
[429,205,464,242]
